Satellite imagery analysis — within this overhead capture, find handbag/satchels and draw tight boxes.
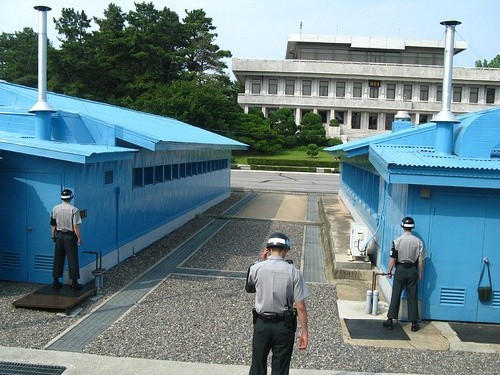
[479,286,492,301]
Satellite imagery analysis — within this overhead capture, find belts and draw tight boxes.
[397,262,416,266]
[257,314,284,321]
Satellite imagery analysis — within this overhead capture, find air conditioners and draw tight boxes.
[350,222,369,257]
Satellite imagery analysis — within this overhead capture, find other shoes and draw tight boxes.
[53,280,62,288]
[411,324,420,332]
[72,282,83,289]
[383,320,393,330]
[57,231,72,234]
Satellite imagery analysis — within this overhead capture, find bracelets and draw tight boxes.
[388,273,391,275]
[52,237,56,239]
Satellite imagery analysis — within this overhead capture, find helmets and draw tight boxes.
[60,190,74,200]
[400,217,415,229]
[266,232,291,250]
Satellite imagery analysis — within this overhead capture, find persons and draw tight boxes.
[245,233,311,375]
[49,189,85,291]
[382,216,424,332]
